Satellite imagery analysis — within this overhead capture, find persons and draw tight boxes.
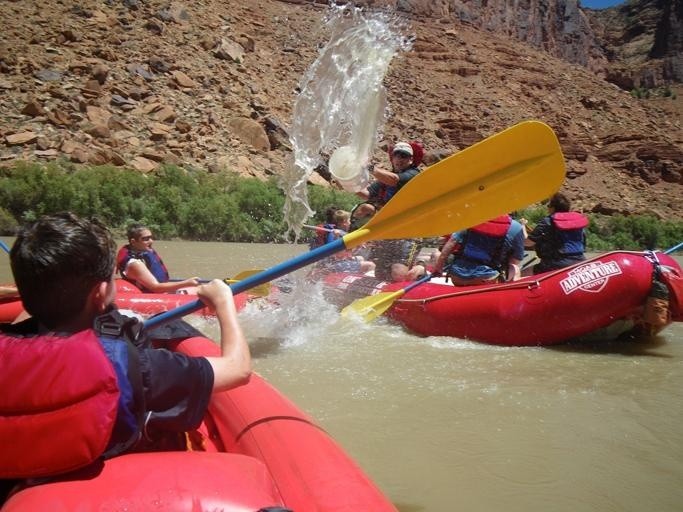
[307,208,341,260]
[531,191,587,275]
[374,236,433,285]
[0,208,254,484]
[343,140,421,232]
[114,223,203,294]
[321,210,358,267]
[431,214,524,288]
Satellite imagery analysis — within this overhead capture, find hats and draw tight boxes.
[392,142,414,157]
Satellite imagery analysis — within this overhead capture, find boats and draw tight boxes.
[0,308,397,512]
[307,249,683,347]
[0,276,249,325]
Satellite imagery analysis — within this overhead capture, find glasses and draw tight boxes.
[393,151,408,158]
[142,236,155,241]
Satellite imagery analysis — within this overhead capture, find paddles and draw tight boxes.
[165,269,272,296]
[340,265,450,325]
[142,119,567,330]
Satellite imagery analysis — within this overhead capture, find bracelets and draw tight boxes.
[367,165,374,172]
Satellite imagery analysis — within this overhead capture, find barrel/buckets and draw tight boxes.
[328,146,370,195]
